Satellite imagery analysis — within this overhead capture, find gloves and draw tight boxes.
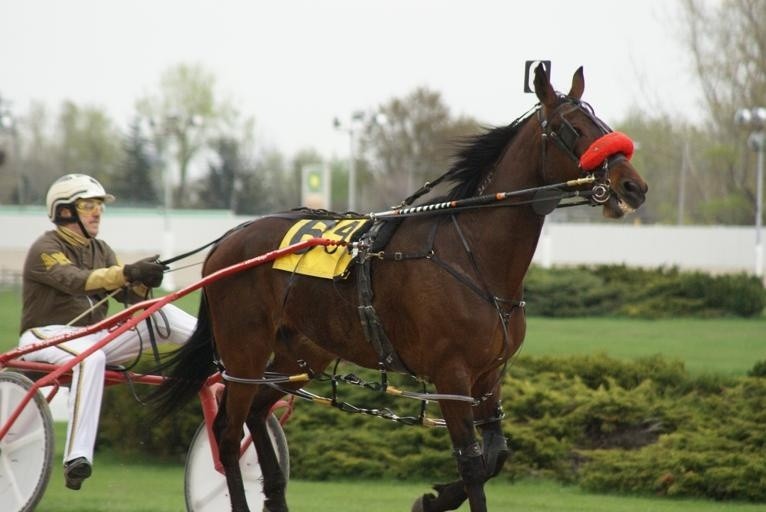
[121,253,169,289]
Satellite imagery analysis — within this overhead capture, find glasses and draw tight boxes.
[73,198,106,218]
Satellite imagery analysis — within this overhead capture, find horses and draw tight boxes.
[125,65,649,512]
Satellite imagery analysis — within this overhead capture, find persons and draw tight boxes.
[11,171,197,490]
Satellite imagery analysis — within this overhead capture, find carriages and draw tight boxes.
[1,58,647,512]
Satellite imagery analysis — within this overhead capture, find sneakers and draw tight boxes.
[62,457,92,492]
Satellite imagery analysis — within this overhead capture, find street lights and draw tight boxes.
[327,109,366,215]
[733,107,765,275]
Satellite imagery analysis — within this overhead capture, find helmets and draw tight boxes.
[44,172,116,223]
